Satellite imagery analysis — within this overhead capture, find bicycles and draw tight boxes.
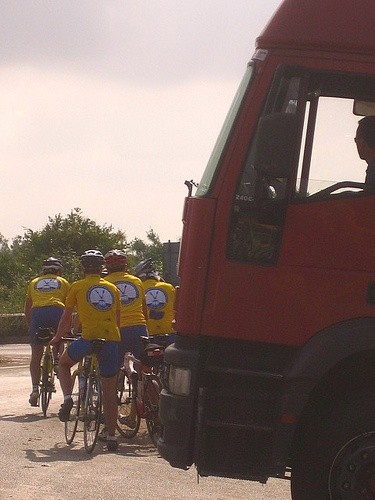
[117,333,167,446]
[57,337,107,453]
[32,327,63,418]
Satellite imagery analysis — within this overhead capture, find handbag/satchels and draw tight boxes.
[143,343,163,367]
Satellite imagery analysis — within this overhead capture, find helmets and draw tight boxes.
[105,249,128,267]
[101,264,108,276]
[41,256,62,270]
[79,249,105,264]
[135,258,155,277]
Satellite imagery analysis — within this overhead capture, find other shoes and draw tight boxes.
[59,398,73,422]
[54,361,59,380]
[107,435,118,451]
[118,414,137,429]
[28,390,39,405]
[98,428,108,441]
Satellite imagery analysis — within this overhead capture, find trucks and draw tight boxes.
[151,0,375,499]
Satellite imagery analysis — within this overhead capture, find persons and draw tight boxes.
[23,256,71,407]
[71,249,176,441]
[49,249,122,452]
[355,115,375,191]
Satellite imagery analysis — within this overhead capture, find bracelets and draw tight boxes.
[71,328,76,336]
